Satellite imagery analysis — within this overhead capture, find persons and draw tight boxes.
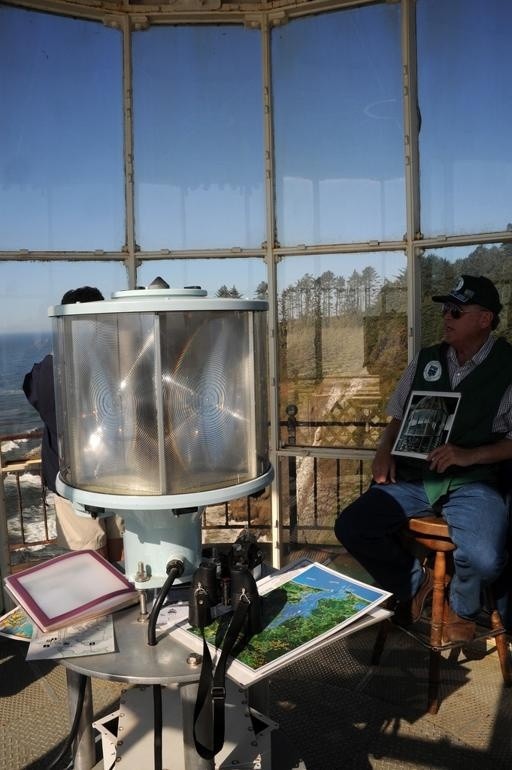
[334,274,512,645]
[23,286,123,571]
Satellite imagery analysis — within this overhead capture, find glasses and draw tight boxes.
[439,301,489,320]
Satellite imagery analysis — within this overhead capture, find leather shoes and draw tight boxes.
[446,608,477,644]
[396,568,432,622]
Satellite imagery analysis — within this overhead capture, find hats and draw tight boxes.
[432,273,503,314]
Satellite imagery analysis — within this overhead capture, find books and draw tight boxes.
[2,549,139,634]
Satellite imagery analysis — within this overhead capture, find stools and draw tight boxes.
[375,513,509,716]
